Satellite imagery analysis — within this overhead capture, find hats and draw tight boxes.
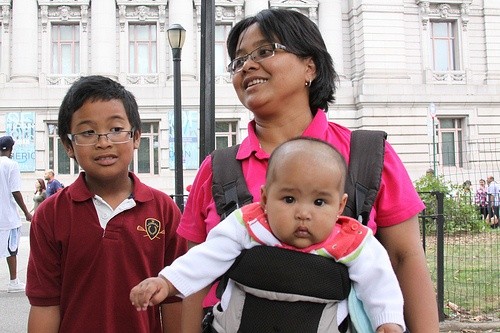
[0,135,15,150]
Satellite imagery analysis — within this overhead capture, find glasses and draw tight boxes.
[67,126,135,145]
[226,42,306,75]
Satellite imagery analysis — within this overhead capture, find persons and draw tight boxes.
[182,9,440,333]
[458,176,499,229]
[131,137,406,333]
[24,76,189,333]
[0,137,66,293]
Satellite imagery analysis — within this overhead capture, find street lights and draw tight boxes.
[165,23,187,213]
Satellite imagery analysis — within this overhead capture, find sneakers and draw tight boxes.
[8,283,26,292]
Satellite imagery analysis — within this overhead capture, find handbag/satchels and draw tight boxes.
[485,205,498,225]
